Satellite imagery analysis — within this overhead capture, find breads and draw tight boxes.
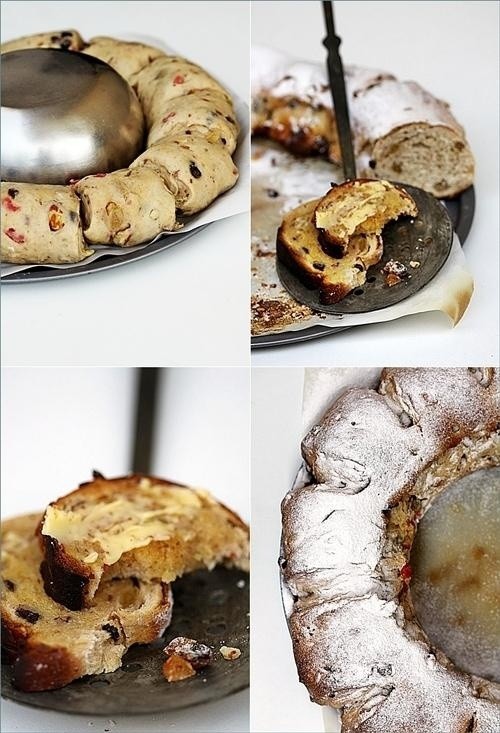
[251,60,475,306]
[277,368,500,732]
[0,27,239,265]
[0,470,251,693]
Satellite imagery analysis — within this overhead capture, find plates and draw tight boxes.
[251,183,476,351]
[1,220,213,282]
[0,565,250,716]
